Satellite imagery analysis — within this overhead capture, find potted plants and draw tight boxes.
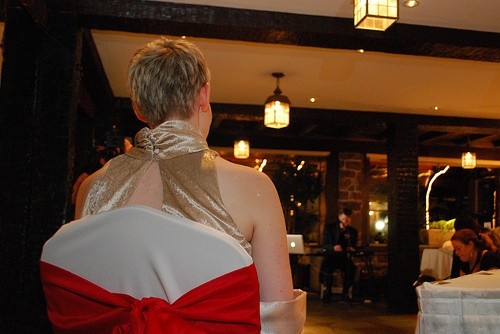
[271,156,325,289]
[423,218,457,246]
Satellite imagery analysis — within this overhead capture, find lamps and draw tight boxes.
[233,130,250,159]
[263,72,291,130]
[461,136,477,168]
[352,0,399,32]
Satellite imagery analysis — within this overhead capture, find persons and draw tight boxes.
[318,206,360,304]
[418,223,429,245]
[68,141,117,209]
[449,214,500,279]
[72,37,310,334]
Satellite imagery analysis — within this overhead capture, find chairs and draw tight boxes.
[40,206,262,334]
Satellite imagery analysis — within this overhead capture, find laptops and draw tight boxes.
[286,234,305,254]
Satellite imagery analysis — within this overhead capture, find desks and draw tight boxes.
[289,250,375,288]
[414,267,500,334]
[420,248,453,281]
